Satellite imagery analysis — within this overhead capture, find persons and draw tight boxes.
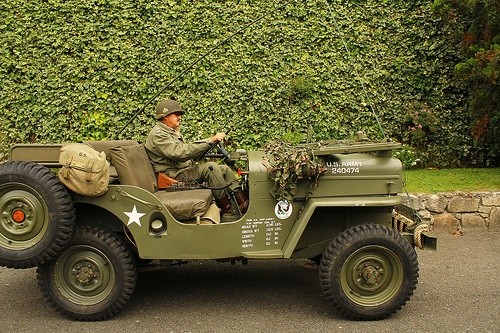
[143,98,248,215]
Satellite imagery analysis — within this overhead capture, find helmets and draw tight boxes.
[155,100,186,120]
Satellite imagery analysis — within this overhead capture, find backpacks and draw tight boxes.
[59,142,110,197]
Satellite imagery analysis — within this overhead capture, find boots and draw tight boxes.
[217,194,247,214]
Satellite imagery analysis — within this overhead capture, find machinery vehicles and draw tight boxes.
[0,131,440,325]
